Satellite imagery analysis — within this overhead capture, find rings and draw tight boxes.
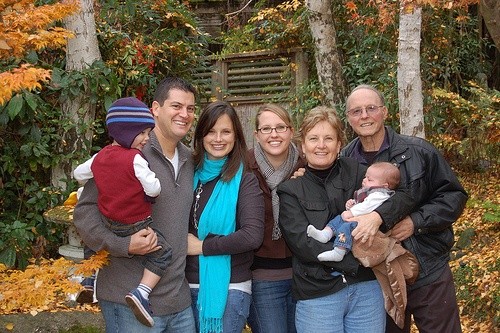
[364,235,369,240]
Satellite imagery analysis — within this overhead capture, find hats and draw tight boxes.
[106,97,156,148]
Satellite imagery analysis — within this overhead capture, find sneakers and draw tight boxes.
[76,278,94,304]
[125,287,154,327]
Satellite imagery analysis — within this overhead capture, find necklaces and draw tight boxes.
[193,183,203,231]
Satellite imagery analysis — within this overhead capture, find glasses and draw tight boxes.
[347,104,383,115]
[257,124,291,134]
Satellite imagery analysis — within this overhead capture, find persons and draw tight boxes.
[276,105,391,333]
[290,84,468,333]
[307,162,400,262]
[246,104,307,333]
[73,98,173,327]
[73,78,196,333]
[184,101,265,333]
